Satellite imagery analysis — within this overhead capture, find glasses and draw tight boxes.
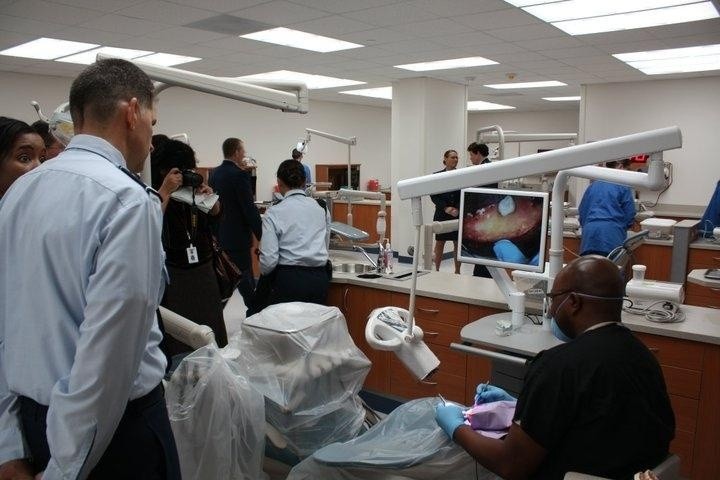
[545,291,575,305]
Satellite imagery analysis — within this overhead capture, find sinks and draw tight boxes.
[333,261,376,275]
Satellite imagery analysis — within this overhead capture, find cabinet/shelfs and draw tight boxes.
[631,331,720,479]
[546,237,720,308]
[332,203,391,246]
[328,283,509,404]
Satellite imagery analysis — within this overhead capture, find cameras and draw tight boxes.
[182,170,206,189]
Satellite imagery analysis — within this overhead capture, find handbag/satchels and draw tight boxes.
[211,235,242,298]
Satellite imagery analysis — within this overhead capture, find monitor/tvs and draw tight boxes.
[454,187,549,273]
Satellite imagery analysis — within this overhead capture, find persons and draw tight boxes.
[257,158,333,304]
[293,148,313,186]
[0,52,184,480]
[459,144,497,280]
[434,253,674,480]
[150,134,262,364]
[576,157,639,257]
[0,117,66,200]
[429,148,465,274]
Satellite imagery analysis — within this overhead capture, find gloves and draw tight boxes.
[475,383,518,406]
[434,401,467,442]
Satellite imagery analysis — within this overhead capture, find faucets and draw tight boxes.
[352,243,378,270]
